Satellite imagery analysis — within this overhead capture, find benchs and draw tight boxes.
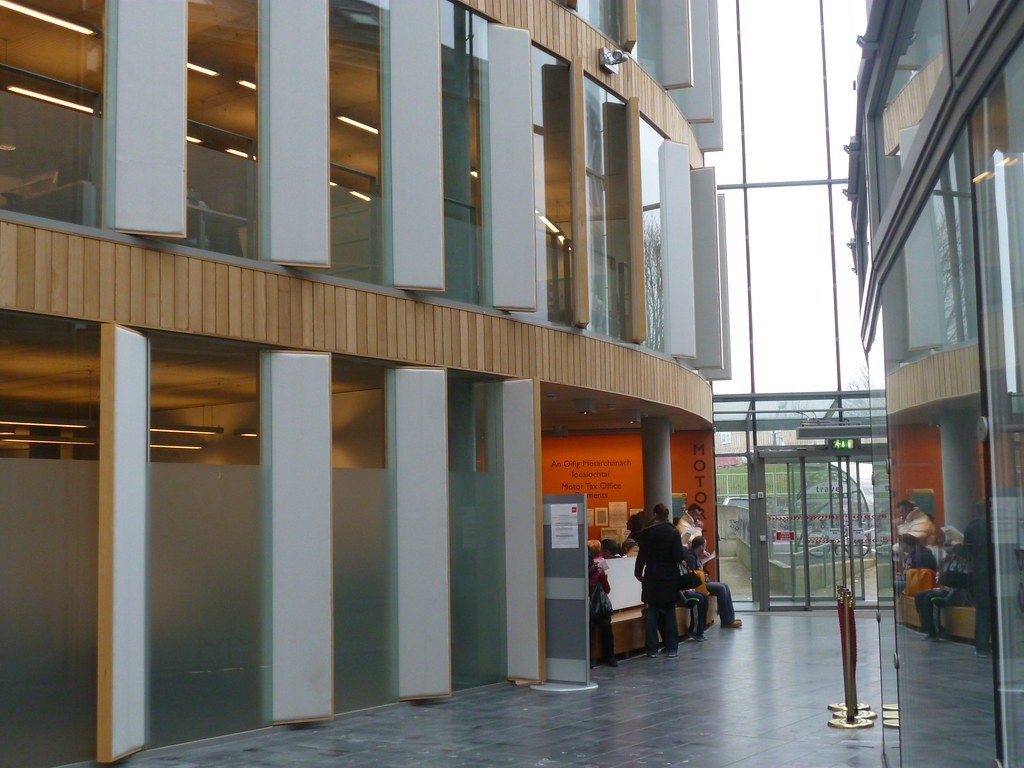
[590,596,714,667]
[901,594,991,643]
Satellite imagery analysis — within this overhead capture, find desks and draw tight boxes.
[187,204,255,250]
[18,180,97,226]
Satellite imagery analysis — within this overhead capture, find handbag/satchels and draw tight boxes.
[901,567,936,597]
[678,561,702,591]
[939,552,966,589]
[589,571,613,616]
[687,559,710,596]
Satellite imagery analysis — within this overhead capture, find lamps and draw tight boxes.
[599,48,629,74]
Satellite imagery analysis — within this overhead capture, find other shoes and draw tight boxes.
[660,648,678,657]
[603,655,618,667]
[721,618,742,628]
[647,647,659,657]
[975,647,993,658]
[920,626,945,641]
[687,631,707,641]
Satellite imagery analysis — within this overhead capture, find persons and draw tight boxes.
[674,504,743,642]
[892,498,993,659]
[588,511,644,668]
[634,504,683,657]
